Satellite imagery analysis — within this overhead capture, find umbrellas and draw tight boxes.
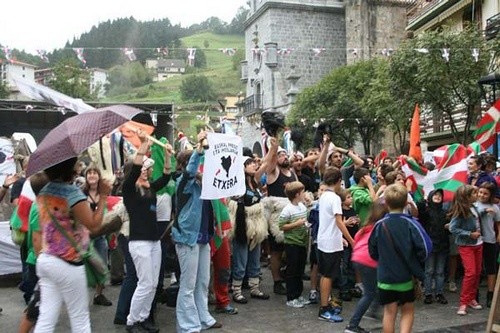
[26,105,145,180]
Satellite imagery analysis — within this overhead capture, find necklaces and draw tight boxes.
[88,192,98,209]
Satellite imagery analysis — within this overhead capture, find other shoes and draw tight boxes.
[302,272,312,280]
[423,281,494,314]
[93,295,112,306]
[126,321,160,333]
[232,295,249,304]
[250,291,270,299]
[286,289,343,322]
[344,323,372,333]
[340,289,362,301]
[274,284,287,295]
[362,311,382,322]
[215,306,238,315]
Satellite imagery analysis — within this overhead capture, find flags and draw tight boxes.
[0,48,480,65]
[399,99,500,204]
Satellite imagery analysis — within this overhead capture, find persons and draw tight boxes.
[0,131,500,333]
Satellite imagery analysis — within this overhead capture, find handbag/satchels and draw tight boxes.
[412,277,424,302]
[87,243,110,275]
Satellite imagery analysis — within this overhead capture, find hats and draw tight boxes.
[128,154,156,171]
[83,161,101,182]
[276,146,287,155]
[175,149,194,166]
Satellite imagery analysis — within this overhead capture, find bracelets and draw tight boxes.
[136,152,144,156]
[165,166,171,171]
[345,151,350,155]
[2,184,9,189]
